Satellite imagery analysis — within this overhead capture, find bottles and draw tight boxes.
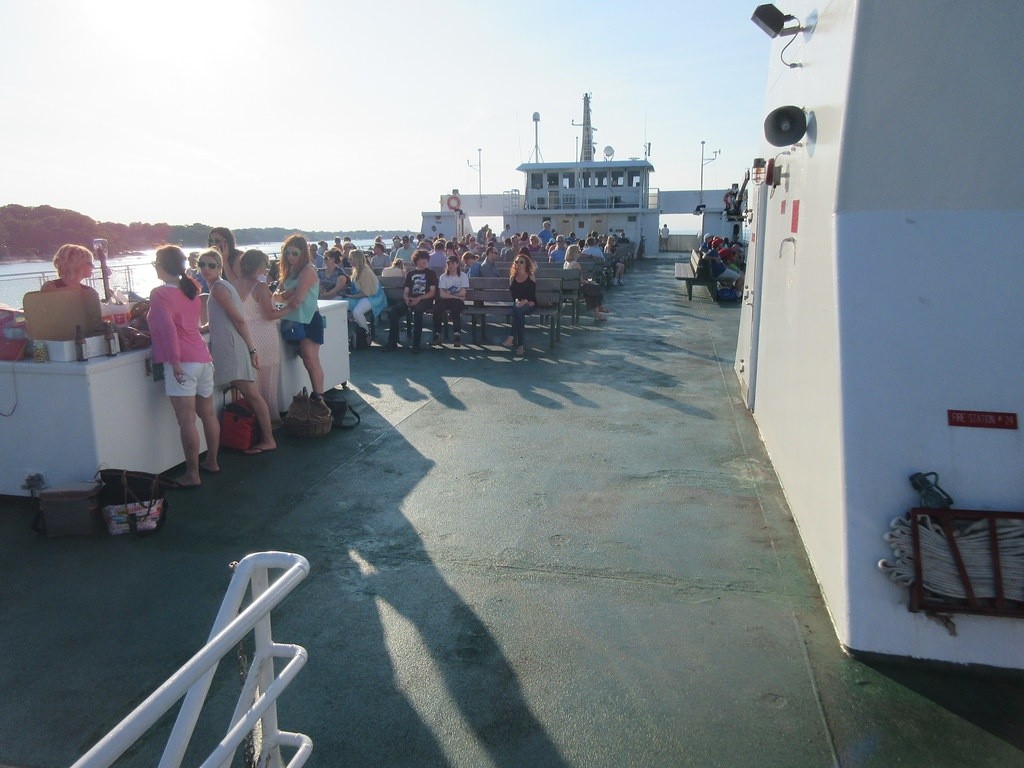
[105,320,117,356]
[909,472,953,509]
[75,324,88,360]
[274,288,284,311]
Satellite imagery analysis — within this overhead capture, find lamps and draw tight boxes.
[751,158,767,186]
[750,3,803,68]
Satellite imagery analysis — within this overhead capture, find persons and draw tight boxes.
[476,221,630,321]
[699,233,746,299]
[502,254,537,355]
[660,224,669,251]
[274,234,324,393]
[196,250,276,456]
[42,244,94,289]
[186,251,200,278]
[195,226,244,293]
[310,232,499,354]
[611,178,618,203]
[147,246,220,488]
[234,249,304,430]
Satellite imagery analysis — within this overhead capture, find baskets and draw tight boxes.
[285,386,334,438]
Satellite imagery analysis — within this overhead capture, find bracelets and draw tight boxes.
[281,290,288,299]
[250,349,256,354]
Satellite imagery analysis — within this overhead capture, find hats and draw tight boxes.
[446,256,458,263]
[703,233,723,249]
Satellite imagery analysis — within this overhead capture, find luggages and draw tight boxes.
[220,384,258,449]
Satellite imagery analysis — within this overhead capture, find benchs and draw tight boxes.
[675,249,701,300]
[700,249,727,302]
[265,235,636,348]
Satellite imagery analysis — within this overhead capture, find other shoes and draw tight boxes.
[595,307,608,322]
[598,277,625,286]
[381,330,524,356]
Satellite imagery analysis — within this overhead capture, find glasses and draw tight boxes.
[152,261,162,268]
[469,238,565,265]
[198,262,219,270]
[83,261,92,267]
[209,238,225,244]
[284,248,301,257]
[323,256,332,261]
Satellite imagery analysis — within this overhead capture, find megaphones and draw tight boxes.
[763,106,807,147]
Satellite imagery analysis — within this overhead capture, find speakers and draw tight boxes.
[751,3,785,39]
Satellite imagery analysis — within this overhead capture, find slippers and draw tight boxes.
[271,423,286,433]
[196,462,219,473]
[163,477,197,491]
[243,446,276,456]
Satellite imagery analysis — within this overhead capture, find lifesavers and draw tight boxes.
[448,196,461,210]
[724,192,736,208]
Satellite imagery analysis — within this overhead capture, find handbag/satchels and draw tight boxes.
[717,286,737,301]
[100,289,133,330]
[95,468,179,536]
[311,389,360,430]
[351,327,373,350]
[282,321,305,342]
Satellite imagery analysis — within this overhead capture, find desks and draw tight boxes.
[0,299,350,498]
